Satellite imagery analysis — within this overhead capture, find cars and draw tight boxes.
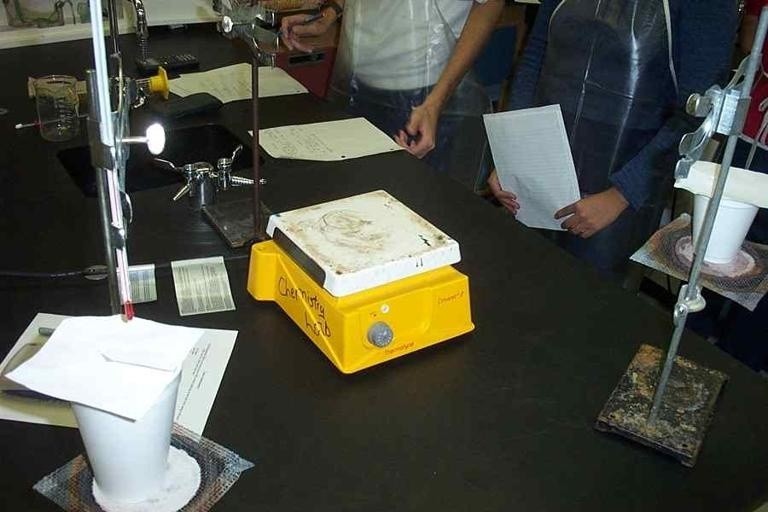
[32,74,81,144]
[693,197,759,263]
[71,366,182,499]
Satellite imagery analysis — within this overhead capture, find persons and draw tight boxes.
[478,0,741,290]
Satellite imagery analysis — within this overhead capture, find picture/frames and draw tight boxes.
[1,21,768,510]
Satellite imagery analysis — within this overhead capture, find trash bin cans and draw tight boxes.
[278,0,511,165]
[328,2,343,19]
